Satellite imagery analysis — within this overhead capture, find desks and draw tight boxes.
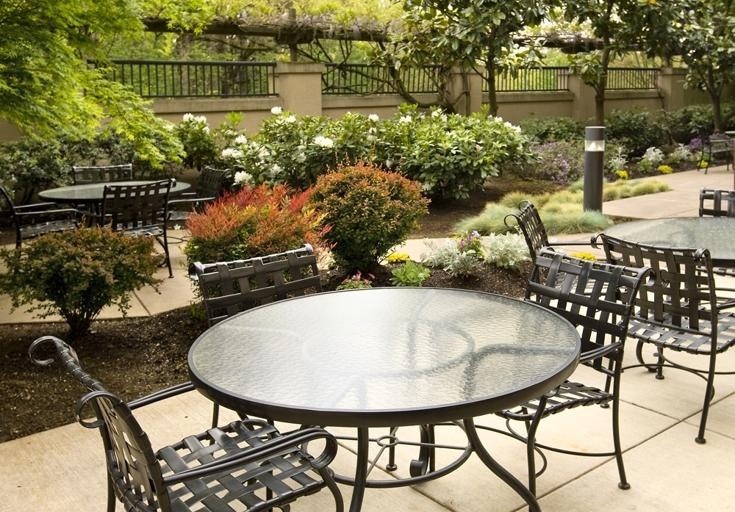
[606,216,735,372]
[40,181,192,265]
[189,286,582,512]
[725,130,735,138]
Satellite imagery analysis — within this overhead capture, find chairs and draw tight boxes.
[695,124,735,174]
[77,179,173,278]
[591,230,735,443]
[71,163,133,185]
[0,186,81,283]
[166,166,238,244]
[502,200,628,375]
[184,241,401,469]
[430,246,655,512]
[699,186,735,218]
[27,336,345,512]
